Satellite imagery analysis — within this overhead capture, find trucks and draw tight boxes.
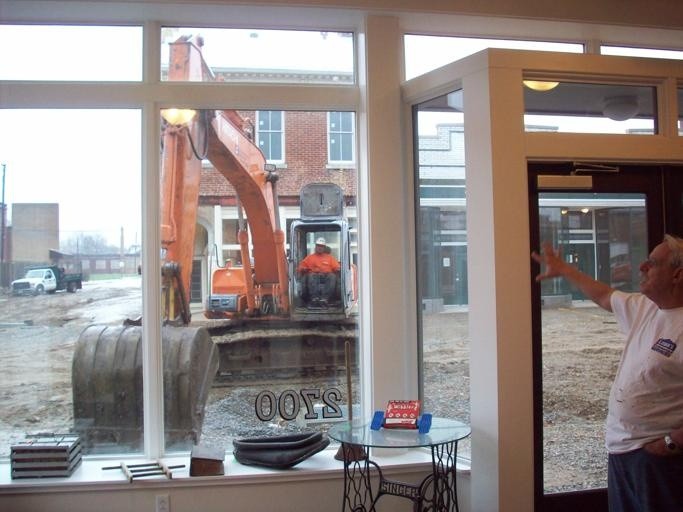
[12,264,84,297]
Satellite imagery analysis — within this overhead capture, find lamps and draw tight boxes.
[522,80,559,91]
[600,94,641,122]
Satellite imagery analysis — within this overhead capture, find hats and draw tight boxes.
[315,237,326,247]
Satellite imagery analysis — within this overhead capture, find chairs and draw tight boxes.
[299,245,342,301]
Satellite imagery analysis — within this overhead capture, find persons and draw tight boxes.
[296,237,340,309]
[531,234,683,512]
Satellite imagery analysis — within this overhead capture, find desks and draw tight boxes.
[326,414,473,511]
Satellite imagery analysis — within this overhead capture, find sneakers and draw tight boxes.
[310,296,329,306]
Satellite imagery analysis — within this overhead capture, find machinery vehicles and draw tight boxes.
[65,35,358,463]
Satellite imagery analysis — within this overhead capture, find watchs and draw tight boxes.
[664,435,683,455]
